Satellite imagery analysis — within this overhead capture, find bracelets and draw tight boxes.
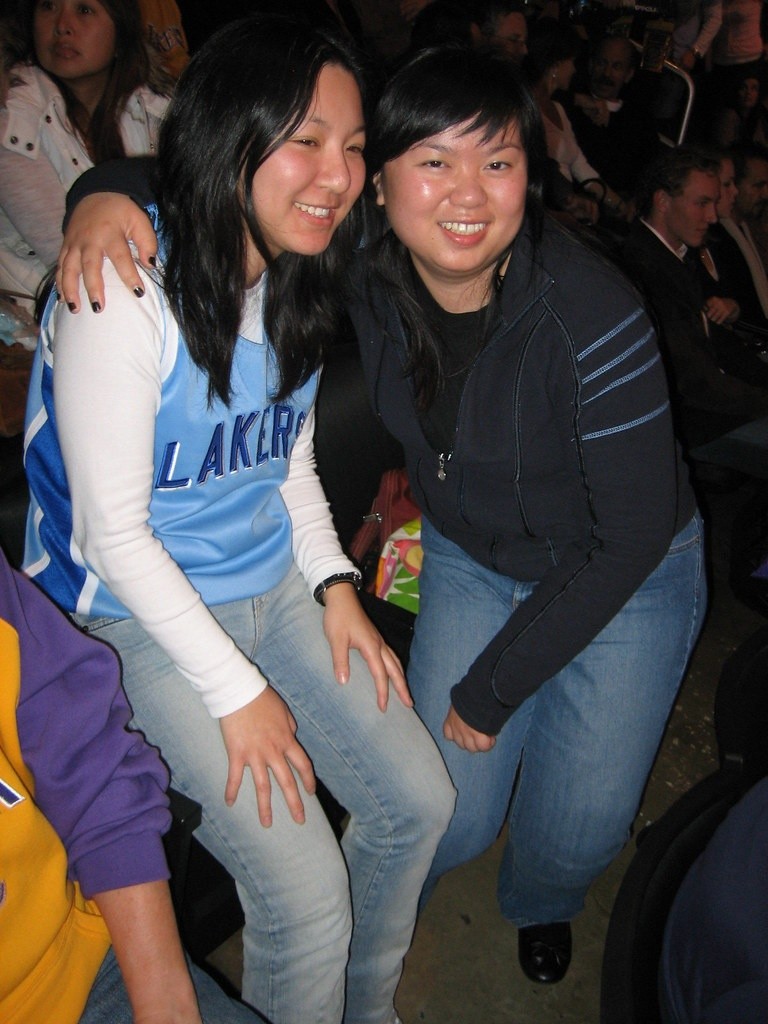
[314,572,363,606]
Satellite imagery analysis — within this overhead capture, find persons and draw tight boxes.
[0,0,768,619]
[55,39,708,985]
[18,13,458,1024]
[0,547,267,1024]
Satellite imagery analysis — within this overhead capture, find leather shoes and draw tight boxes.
[518,920,572,984]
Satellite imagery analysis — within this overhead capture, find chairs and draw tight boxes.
[599,621,766,1024]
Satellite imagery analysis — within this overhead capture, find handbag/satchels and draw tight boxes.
[348,467,421,596]
[705,307,768,386]
